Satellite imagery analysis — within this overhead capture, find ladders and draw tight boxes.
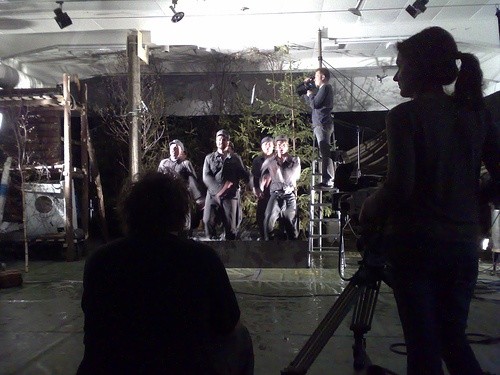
[308,132,346,269]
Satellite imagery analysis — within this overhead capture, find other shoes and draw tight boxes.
[315,183,333,188]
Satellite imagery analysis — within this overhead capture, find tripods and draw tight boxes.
[281,231,391,375]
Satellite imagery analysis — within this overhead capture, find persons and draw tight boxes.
[202,129,247,241]
[260,136,302,242]
[250,137,279,238]
[303,68,337,190]
[157,138,203,230]
[73,167,255,375]
[357,25,500,375]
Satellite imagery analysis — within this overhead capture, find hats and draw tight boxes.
[169,139,185,151]
[261,136,274,144]
[276,135,289,143]
[216,129,231,140]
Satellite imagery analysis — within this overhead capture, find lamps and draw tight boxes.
[54,0,72,30]
[406,1,430,18]
[169,0,184,24]
[376,73,389,83]
[348,0,365,15]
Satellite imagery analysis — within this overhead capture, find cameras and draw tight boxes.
[296,80,316,97]
